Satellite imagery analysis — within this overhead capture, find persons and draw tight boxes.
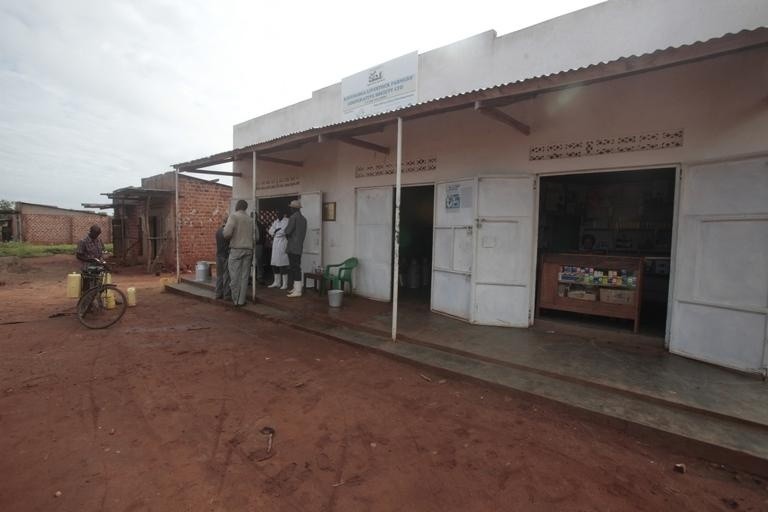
[267,210,290,290]
[582,234,595,250]
[223,199,259,307]
[215,216,232,301]
[250,212,266,285]
[76,225,122,305]
[284,200,307,298]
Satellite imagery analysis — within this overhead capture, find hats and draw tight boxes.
[288,200,302,208]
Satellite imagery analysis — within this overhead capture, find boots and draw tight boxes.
[287,281,301,297]
[268,274,281,288]
[279,274,288,289]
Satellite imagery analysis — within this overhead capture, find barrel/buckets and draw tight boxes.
[326,289,344,308]
[66,271,82,299]
[194,260,212,284]
[101,294,116,310]
[159,277,171,293]
[102,273,113,293]
[127,287,137,307]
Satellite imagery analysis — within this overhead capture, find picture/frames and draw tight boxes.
[322,201,336,222]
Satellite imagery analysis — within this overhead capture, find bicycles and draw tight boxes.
[73,256,129,331]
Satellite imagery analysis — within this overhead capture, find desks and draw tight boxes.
[302,271,333,295]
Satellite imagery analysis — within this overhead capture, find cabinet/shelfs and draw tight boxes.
[642,249,671,305]
[539,245,646,335]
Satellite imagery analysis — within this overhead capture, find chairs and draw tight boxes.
[320,255,358,298]
